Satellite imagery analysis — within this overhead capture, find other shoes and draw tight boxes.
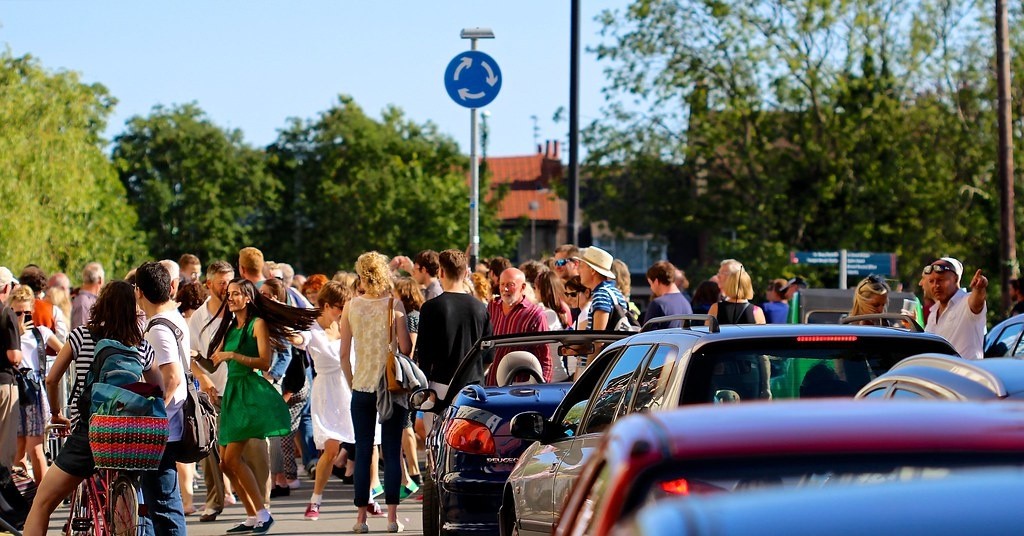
[183,467,425,516]
[331,462,354,485]
[199,507,224,522]
[387,521,404,533]
[286,479,300,490]
[353,523,368,533]
[270,483,291,498]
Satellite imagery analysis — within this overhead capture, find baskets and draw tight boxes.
[88,414,170,471]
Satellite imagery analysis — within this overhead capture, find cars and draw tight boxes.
[854,351,1024,403]
[495,313,965,536]
[769,288,924,399]
[554,396,1024,536]
[982,314,1024,358]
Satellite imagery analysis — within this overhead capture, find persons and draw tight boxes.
[464,244,694,385]
[834,273,917,383]
[1007,278,1024,318]
[688,256,810,402]
[0,246,497,536]
[918,257,989,360]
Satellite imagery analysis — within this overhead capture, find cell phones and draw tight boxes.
[24,315,32,330]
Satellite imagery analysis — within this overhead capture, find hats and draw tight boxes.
[0,266,19,287]
[572,245,616,280]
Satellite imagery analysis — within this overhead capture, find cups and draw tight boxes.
[901,299,917,329]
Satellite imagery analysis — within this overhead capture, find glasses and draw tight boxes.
[923,264,957,275]
[554,257,574,267]
[14,311,32,317]
[274,277,285,281]
[333,303,344,310]
[858,273,884,292]
[564,291,580,298]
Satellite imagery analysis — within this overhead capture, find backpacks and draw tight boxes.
[592,287,643,360]
[76,325,147,420]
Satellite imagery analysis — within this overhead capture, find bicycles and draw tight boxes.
[46,420,146,536]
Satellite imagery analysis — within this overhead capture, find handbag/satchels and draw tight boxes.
[16,367,40,406]
[386,352,412,394]
[175,379,218,464]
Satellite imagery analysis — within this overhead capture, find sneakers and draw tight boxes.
[368,503,385,518]
[251,515,274,535]
[303,503,320,520]
[226,523,255,534]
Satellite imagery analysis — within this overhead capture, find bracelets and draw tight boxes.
[241,355,245,362]
[249,357,252,366]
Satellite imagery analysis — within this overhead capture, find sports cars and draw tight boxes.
[409,329,639,536]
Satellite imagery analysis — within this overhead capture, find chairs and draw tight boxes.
[497,351,546,387]
